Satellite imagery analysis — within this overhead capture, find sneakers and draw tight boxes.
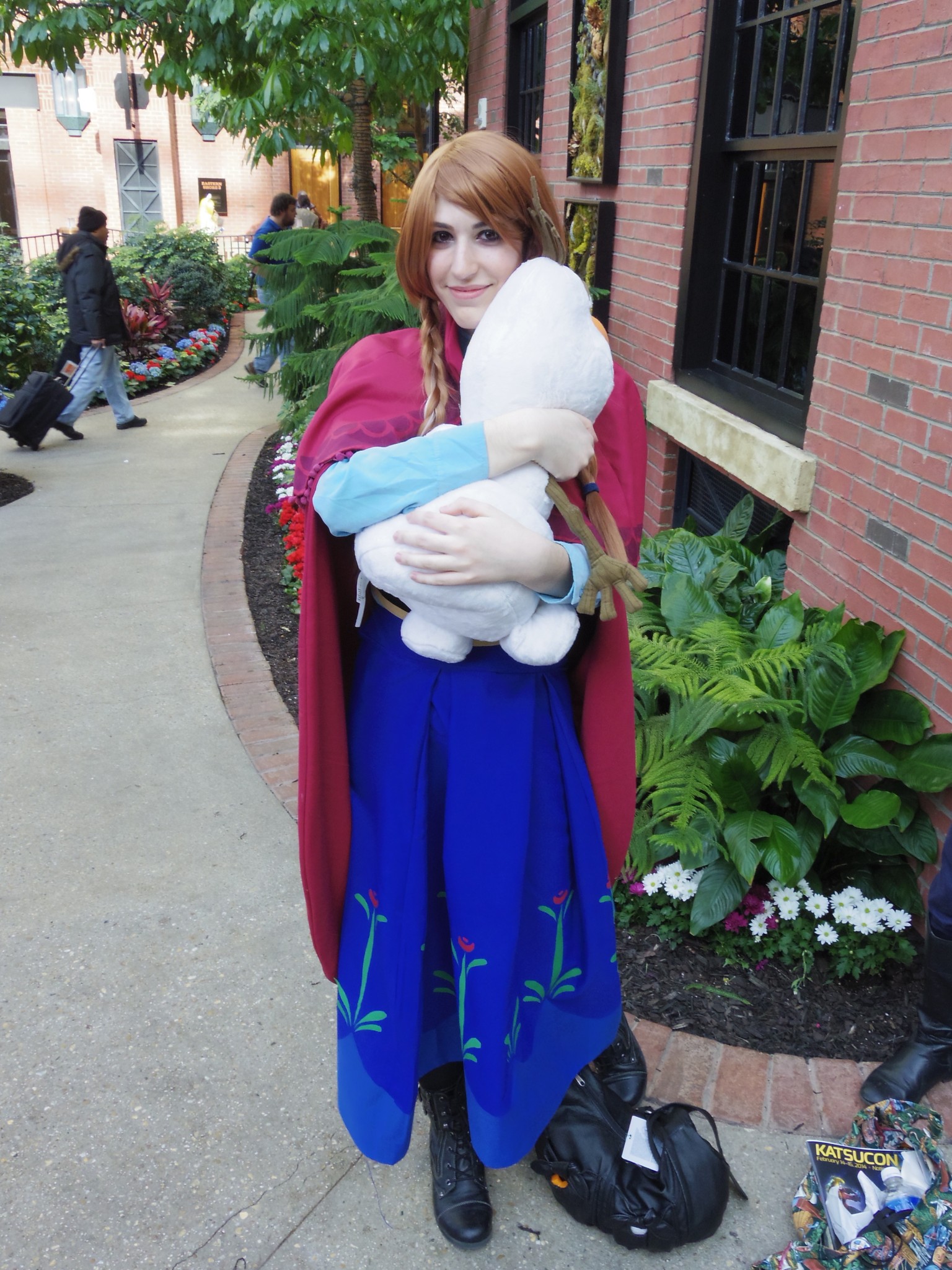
[117,415,147,429]
[53,420,83,440]
[245,360,268,388]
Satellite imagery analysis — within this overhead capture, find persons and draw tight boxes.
[292,130,654,1249]
[241,192,299,382]
[292,191,319,230]
[860,826,951,1112]
[48,205,148,440]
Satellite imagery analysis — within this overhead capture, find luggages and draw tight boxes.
[0,339,104,452]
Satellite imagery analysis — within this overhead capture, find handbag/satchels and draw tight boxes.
[531,1062,749,1254]
[750,1099,952,1270]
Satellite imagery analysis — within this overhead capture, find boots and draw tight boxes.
[592,1007,648,1118]
[418,1068,493,1249]
[858,908,952,1106]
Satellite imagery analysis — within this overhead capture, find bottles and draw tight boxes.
[881,1166,927,1223]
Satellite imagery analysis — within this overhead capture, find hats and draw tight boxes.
[77,206,107,232]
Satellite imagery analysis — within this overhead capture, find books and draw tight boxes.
[805,1139,934,1252]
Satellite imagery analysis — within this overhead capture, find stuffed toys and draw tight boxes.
[352,255,648,670]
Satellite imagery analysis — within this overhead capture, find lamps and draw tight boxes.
[50,57,91,136]
[190,75,224,141]
